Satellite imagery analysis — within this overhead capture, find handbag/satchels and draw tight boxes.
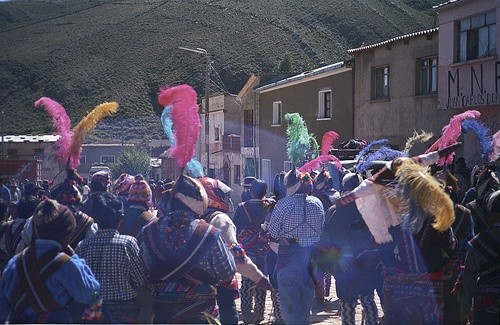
[383,266,465,325]
[218,279,239,299]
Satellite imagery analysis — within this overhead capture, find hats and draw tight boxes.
[90,171,110,190]
[56,178,85,203]
[170,174,208,218]
[241,175,256,187]
[284,165,334,196]
[342,172,363,192]
[112,173,152,207]
[33,198,76,245]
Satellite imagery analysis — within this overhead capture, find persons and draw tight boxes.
[0,131,500,324]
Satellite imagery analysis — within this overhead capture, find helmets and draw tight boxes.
[197,177,233,213]
